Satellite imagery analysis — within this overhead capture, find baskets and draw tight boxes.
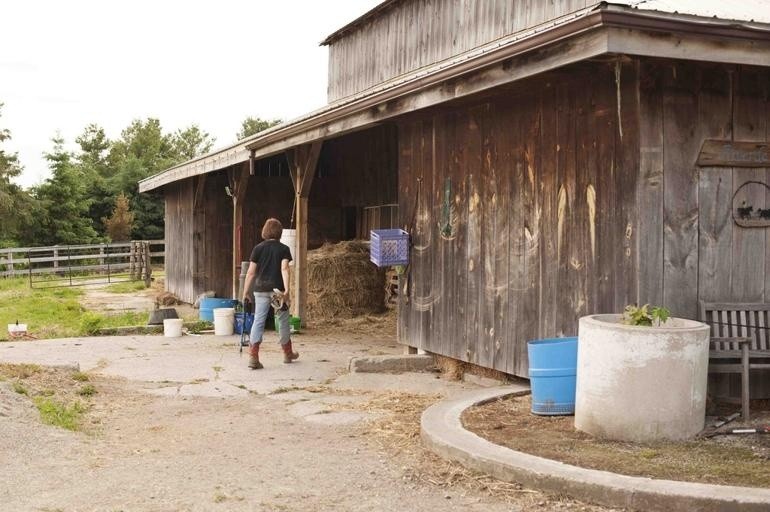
[370,228,410,268]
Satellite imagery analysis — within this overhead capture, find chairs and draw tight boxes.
[701,299,769,421]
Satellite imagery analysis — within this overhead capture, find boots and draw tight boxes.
[280,339,299,364]
[247,341,264,368]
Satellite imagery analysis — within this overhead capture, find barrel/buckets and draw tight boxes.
[213,308,235,335]
[288,316,300,333]
[162,318,184,337]
[526,337,578,416]
[199,297,239,322]
[279,228,296,267]
[273,314,293,331]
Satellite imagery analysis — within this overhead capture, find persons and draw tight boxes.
[242,218,299,369]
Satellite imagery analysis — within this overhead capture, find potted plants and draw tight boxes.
[573,304,711,442]
